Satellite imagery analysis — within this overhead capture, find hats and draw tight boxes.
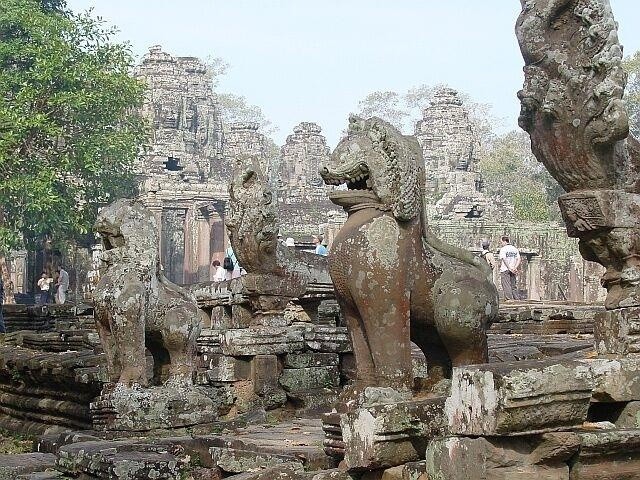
[483,241,489,248]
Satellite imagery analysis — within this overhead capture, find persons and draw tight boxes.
[313,233,327,256]
[38,271,53,303]
[480,239,496,270]
[54,266,70,304]
[212,259,226,282]
[500,236,521,300]
[223,239,241,281]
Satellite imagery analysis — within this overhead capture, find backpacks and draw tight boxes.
[480,252,488,259]
[223,257,234,271]
